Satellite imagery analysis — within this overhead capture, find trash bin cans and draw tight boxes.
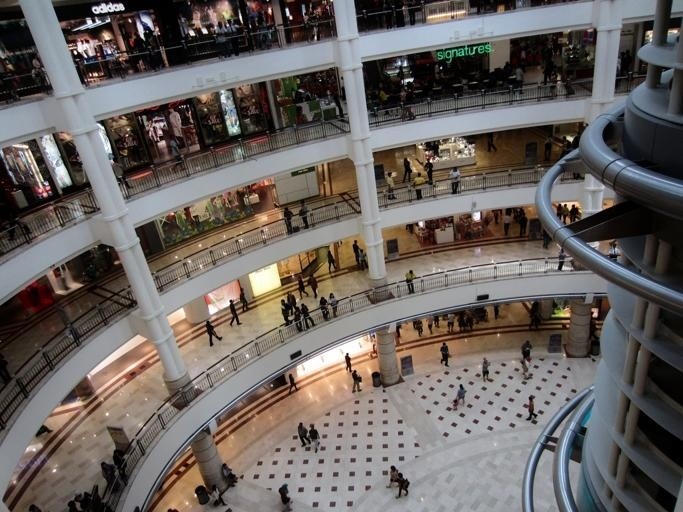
[590,334,599,357]
[372,372,381,387]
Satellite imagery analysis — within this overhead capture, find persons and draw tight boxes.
[537,33,633,343]
